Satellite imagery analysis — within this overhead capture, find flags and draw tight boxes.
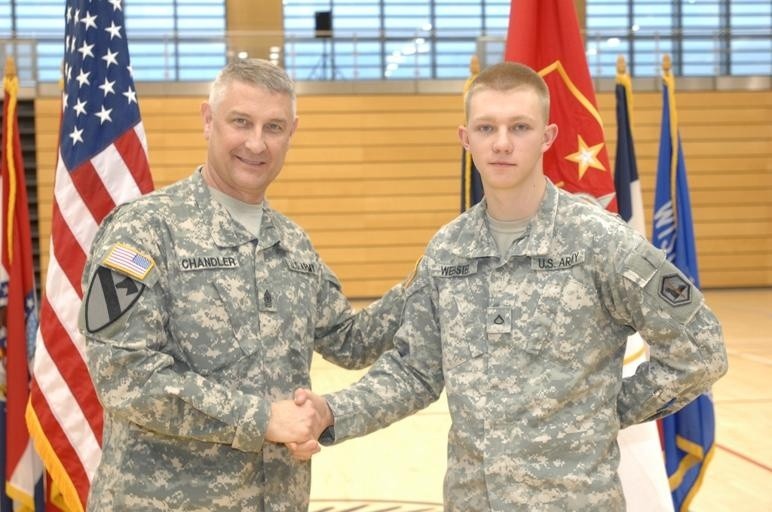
[23,0,157,511]
[502,2,677,511]
[613,74,650,246]
[0,61,44,512]
[649,50,718,512]
[459,60,492,215]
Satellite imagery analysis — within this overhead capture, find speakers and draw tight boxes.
[315,10,333,38]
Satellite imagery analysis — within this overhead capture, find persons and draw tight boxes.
[285,60,727,512]
[76,55,422,512]
